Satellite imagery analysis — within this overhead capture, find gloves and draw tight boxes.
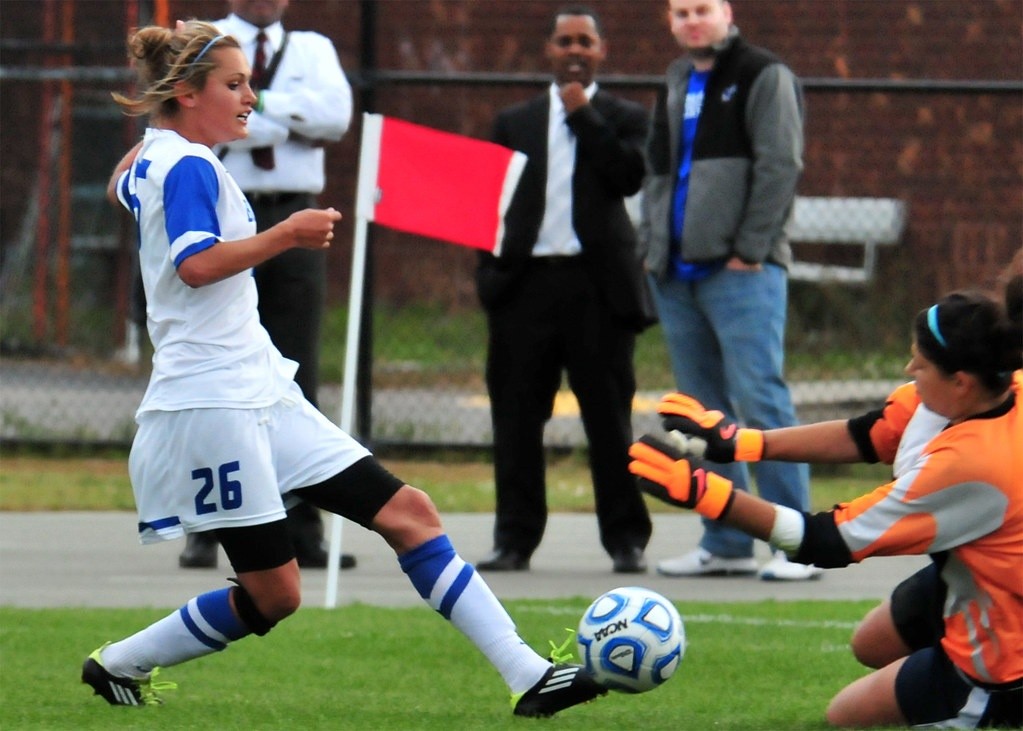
[652,393,764,468]
[619,433,734,520]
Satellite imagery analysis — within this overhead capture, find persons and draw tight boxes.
[180,0,359,568]
[634,0,822,581]
[83,20,609,719]
[628,295,1023,731]
[473,7,654,576]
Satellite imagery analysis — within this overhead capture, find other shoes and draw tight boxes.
[302,546,357,569]
[477,545,533,573]
[656,549,760,578]
[609,546,647,575]
[757,549,825,581]
[177,533,219,570]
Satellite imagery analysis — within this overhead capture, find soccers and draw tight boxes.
[578,586,687,694]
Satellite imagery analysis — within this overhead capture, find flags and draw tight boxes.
[364,113,528,257]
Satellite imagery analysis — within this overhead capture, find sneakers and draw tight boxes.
[508,626,619,721]
[81,637,180,706]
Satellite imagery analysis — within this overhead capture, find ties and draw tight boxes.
[248,28,281,178]
